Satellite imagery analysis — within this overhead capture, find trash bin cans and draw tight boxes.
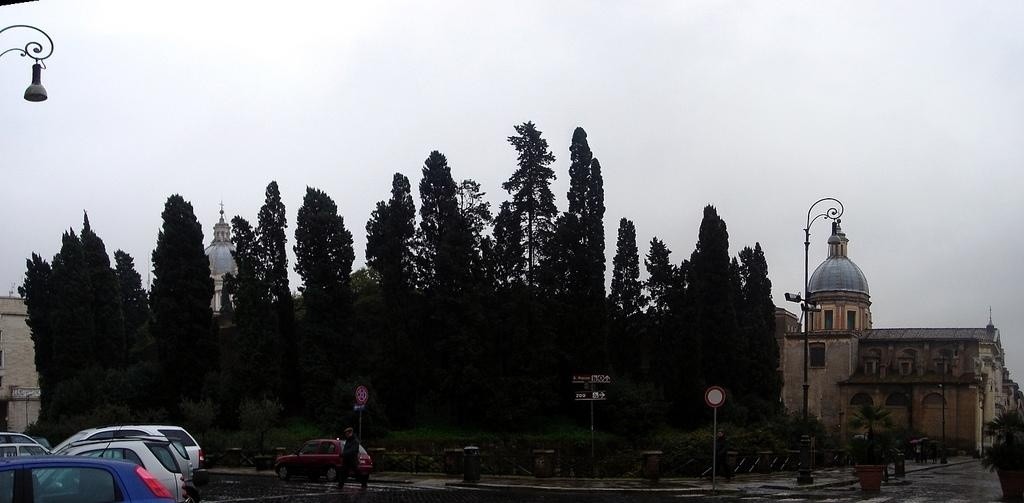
[463,446,481,480]
[532,450,555,478]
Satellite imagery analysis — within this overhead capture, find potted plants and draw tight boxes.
[845,392,904,494]
[235,391,287,472]
[975,403,1024,502]
[186,392,218,470]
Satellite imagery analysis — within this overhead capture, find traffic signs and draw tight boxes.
[574,389,608,400]
[572,374,611,385]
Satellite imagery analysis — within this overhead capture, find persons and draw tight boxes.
[329,425,360,491]
[909,436,948,464]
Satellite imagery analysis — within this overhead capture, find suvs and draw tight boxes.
[0,424,204,502]
[275,438,371,482]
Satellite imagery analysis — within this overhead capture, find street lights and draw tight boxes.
[786,195,844,484]
[0,20,54,101]
[980,377,995,457]
[941,340,963,464]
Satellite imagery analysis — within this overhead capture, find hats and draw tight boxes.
[344,428,353,432]
